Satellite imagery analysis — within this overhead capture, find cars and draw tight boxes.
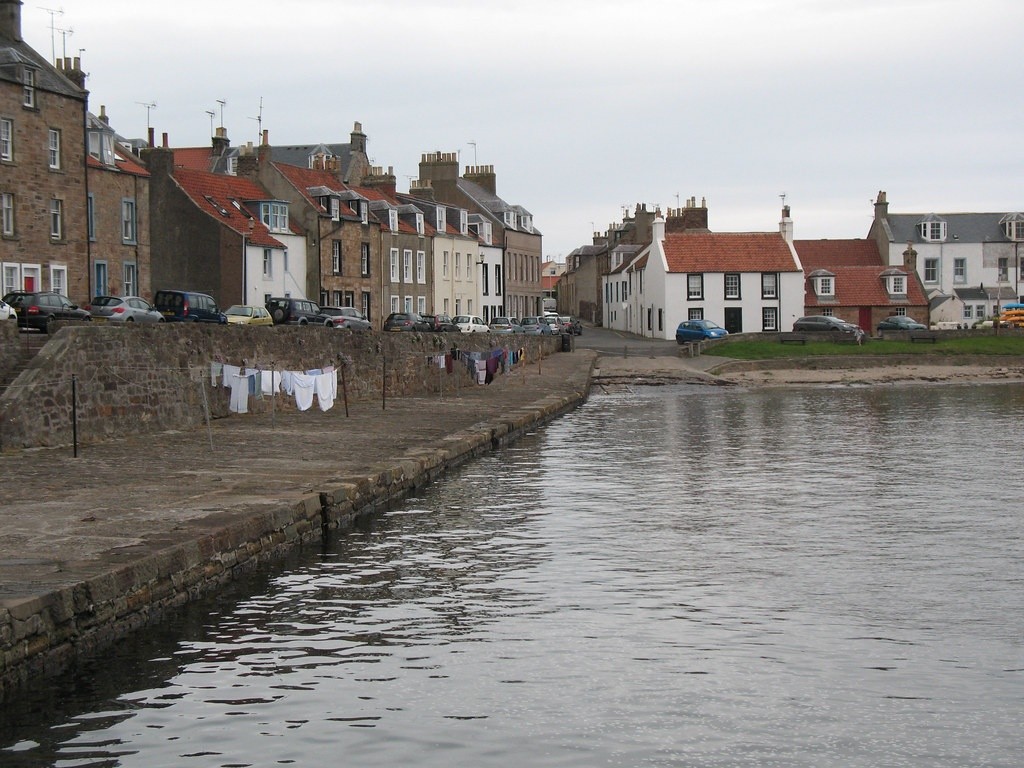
[82,295,167,323]
[519,316,553,336]
[451,315,490,333]
[383,312,432,333]
[543,310,565,336]
[418,313,460,333]
[0,298,18,324]
[558,316,583,336]
[222,304,274,327]
[875,315,928,338]
[488,316,526,333]
[676,319,729,345]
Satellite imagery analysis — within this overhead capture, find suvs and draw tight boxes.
[264,297,321,325]
[153,289,229,326]
[319,305,373,331]
[2,290,93,334]
[792,315,861,334]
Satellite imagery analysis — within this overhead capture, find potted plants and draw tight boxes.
[957,324,961,329]
[992,317,999,328]
[972,324,976,329]
[964,323,968,329]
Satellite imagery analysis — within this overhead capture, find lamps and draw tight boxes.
[476,252,485,265]
[248,217,254,233]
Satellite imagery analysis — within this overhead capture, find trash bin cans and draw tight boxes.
[562,333,571,352]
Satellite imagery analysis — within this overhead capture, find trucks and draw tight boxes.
[929,321,959,331]
